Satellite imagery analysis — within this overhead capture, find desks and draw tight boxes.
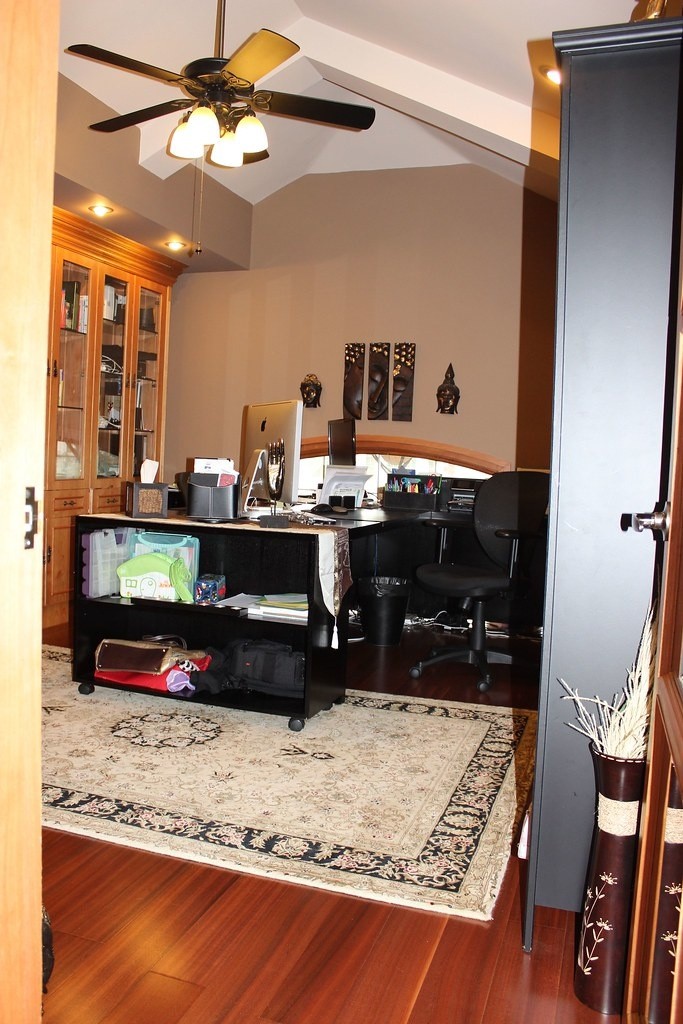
[237,507,521,625]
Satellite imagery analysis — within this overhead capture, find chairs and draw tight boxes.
[407,472,550,693]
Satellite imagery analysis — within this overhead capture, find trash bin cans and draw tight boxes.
[357,576,413,646]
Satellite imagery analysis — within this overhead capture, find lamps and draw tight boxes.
[170,99,271,255]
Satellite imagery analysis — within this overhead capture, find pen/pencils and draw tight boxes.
[383,477,442,494]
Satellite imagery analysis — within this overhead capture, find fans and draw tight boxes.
[66,0,376,165]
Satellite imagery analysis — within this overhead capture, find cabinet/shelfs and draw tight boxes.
[90,221,189,515]
[42,205,91,518]
[43,518,72,629]
[73,512,381,732]
[521,19,683,954]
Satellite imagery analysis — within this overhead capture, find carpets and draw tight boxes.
[42,643,538,921]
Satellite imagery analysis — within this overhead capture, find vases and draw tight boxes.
[573,740,648,1016]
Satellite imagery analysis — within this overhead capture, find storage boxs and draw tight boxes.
[382,490,439,512]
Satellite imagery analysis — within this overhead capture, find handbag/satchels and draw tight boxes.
[92,633,305,703]
